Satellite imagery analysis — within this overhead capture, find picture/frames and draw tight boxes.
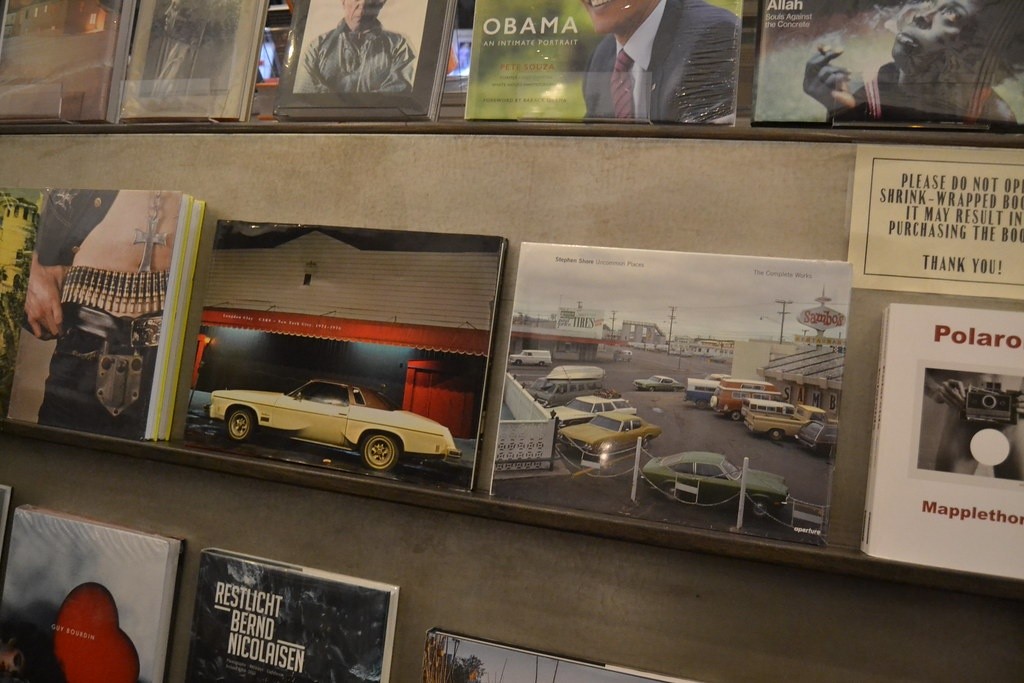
[272,0,458,121]
[106,0,270,123]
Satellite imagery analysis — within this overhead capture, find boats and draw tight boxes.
[546,365,605,380]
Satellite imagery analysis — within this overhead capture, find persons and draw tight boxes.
[293,0,416,94]
[578,0,742,124]
[0,622,41,683]
[924,367,1024,482]
[24,186,182,439]
[802,0,1024,127]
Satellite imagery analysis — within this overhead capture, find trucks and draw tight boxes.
[684,377,722,410]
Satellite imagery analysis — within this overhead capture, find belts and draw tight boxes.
[70,305,164,349]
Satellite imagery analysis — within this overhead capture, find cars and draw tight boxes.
[551,395,636,430]
[643,450,793,521]
[557,411,661,459]
[794,420,838,458]
[633,375,686,393]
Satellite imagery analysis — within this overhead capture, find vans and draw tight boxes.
[509,350,552,366]
[710,373,731,382]
[709,379,782,422]
[740,398,796,417]
[527,375,602,409]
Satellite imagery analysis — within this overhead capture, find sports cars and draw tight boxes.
[203,377,463,472]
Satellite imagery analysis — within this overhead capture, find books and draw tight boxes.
[0,0,1024,683]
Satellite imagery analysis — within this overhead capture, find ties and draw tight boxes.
[609,49,636,120]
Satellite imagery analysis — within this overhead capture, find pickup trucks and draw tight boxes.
[743,404,838,442]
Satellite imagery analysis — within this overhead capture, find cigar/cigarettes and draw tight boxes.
[816,45,831,62]
